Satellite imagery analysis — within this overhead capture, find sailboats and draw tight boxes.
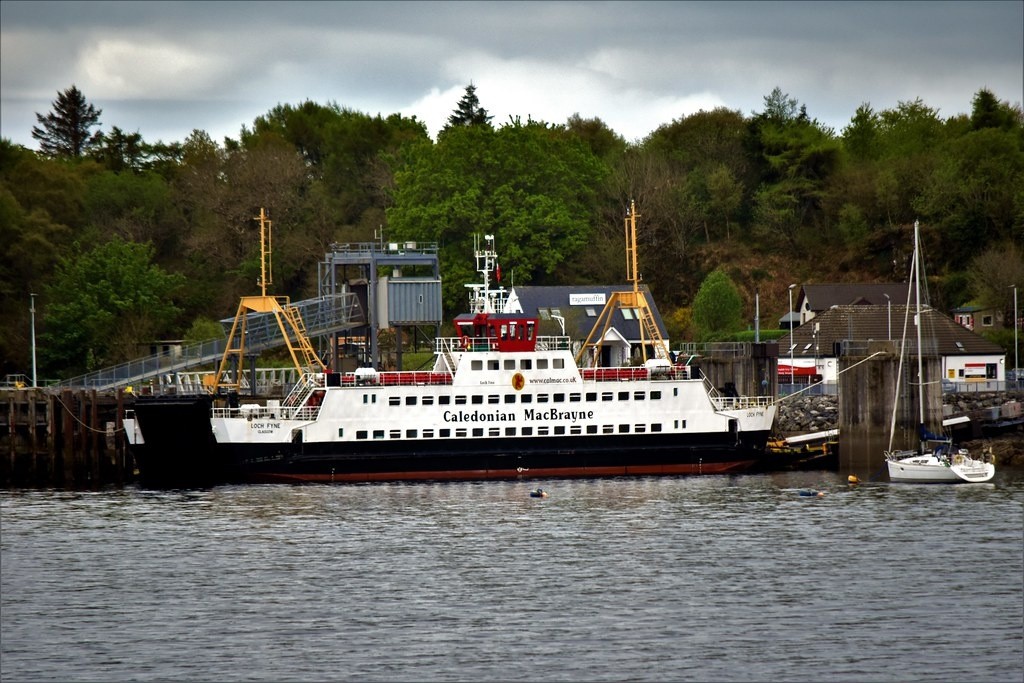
[885,220,995,483]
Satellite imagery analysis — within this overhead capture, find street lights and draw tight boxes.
[30,293,38,387]
[788,284,796,394]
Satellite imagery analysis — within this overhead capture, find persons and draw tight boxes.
[1007,369,1016,388]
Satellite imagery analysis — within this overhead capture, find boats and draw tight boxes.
[116,199,778,487]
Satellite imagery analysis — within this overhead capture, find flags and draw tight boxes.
[496,260,501,283]
